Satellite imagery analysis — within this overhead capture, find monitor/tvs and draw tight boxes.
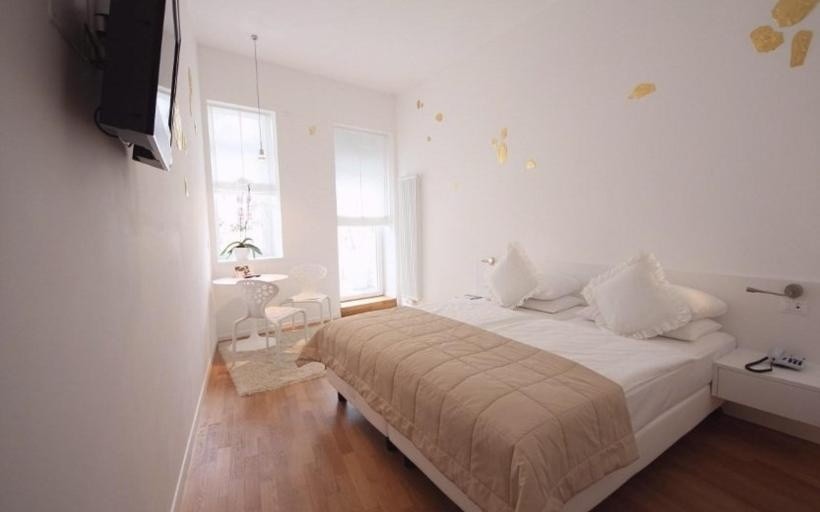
[95,1,182,173]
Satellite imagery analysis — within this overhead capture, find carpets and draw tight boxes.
[218,323,329,398]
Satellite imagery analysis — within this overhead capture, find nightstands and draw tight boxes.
[711,349,820,446]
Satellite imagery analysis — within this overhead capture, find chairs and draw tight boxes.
[227,281,308,372]
[278,263,332,341]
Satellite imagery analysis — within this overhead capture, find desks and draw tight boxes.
[213,274,288,353]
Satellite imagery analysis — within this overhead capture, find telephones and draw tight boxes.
[769,342,807,371]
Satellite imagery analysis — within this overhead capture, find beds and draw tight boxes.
[298,263,736,512]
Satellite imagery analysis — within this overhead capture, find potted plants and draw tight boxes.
[220,237,263,279]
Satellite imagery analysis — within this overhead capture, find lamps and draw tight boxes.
[480,257,495,265]
[250,34,268,161]
[746,284,802,298]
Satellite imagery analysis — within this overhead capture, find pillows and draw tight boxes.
[660,320,724,342]
[513,288,589,314]
[530,267,590,300]
[588,259,693,341]
[577,284,729,320]
[486,243,541,310]
